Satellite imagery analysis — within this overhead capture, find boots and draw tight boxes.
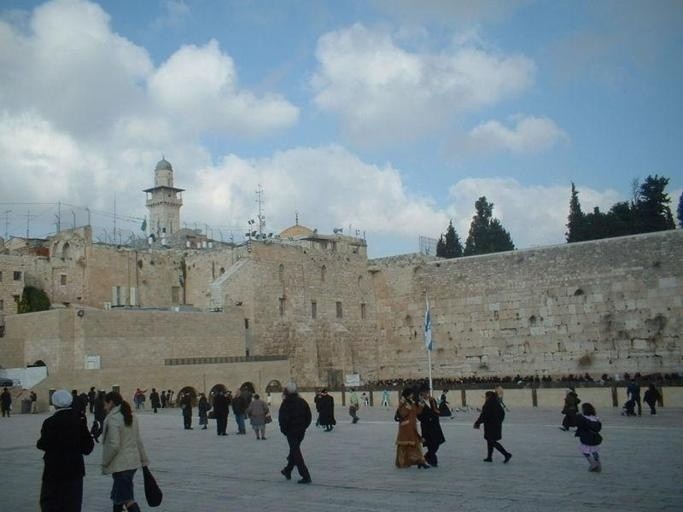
[112,502,124,512]
[126,502,139,512]
[586,455,601,471]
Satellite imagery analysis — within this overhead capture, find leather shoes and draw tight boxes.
[185,426,193,429]
[222,432,227,436]
[201,425,206,428]
[297,477,310,483]
[262,437,267,440]
[503,453,512,462]
[258,437,260,440]
[217,432,220,435]
[243,432,246,434]
[236,432,242,435]
[559,427,565,431]
[484,458,491,461]
[281,467,290,479]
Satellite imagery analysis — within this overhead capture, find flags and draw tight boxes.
[423,296,433,351]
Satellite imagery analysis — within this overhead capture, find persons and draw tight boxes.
[100,391,149,512]
[278,382,313,483]
[621,399,636,416]
[88,387,95,412]
[641,383,663,416]
[558,386,580,431]
[313,389,326,415]
[348,386,359,425]
[399,387,440,468]
[207,390,231,419]
[134,389,146,409]
[316,389,336,432]
[212,387,228,435]
[29,390,39,414]
[245,395,269,440]
[394,382,446,466]
[198,394,208,429]
[0,387,11,417]
[438,389,454,417]
[95,391,107,442]
[72,392,89,415]
[161,390,167,406]
[232,390,248,434]
[149,388,160,413]
[626,378,642,415]
[575,402,603,471]
[474,392,513,463]
[36,390,94,512]
[179,391,193,430]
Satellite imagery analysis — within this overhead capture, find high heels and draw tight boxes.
[417,463,429,469]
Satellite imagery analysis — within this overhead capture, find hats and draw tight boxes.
[287,382,296,393]
[52,390,74,407]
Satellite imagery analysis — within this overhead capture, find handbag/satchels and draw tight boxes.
[206,402,212,411]
[141,464,162,507]
[265,411,272,423]
